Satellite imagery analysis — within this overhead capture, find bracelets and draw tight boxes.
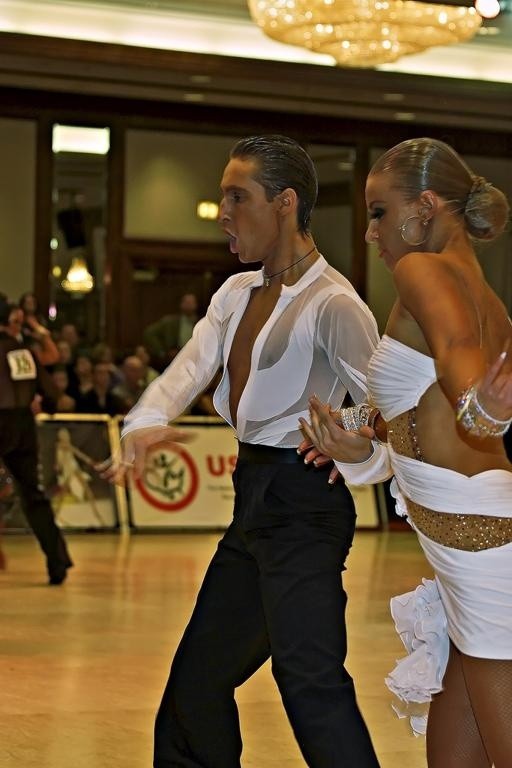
[356,403,369,426]
[364,407,375,426]
[346,408,358,431]
[340,409,350,431]
[469,393,510,436]
[369,408,381,442]
[458,394,488,439]
[35,326,45,335]
[360,408,366,424]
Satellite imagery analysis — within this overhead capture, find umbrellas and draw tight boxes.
[50,557,74,584]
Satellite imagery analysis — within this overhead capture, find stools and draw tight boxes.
[233,440,305,466]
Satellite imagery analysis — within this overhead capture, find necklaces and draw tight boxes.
[264,247,315,287]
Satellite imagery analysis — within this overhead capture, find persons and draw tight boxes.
[17,293,130,497]
[2,293,74,588]
[102,134,396,768]
[296,138,510,767]
[113,293,223,416]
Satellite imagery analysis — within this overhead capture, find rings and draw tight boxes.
[122,461,134,468]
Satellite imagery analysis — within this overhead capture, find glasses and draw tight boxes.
[245,0,483,70]
